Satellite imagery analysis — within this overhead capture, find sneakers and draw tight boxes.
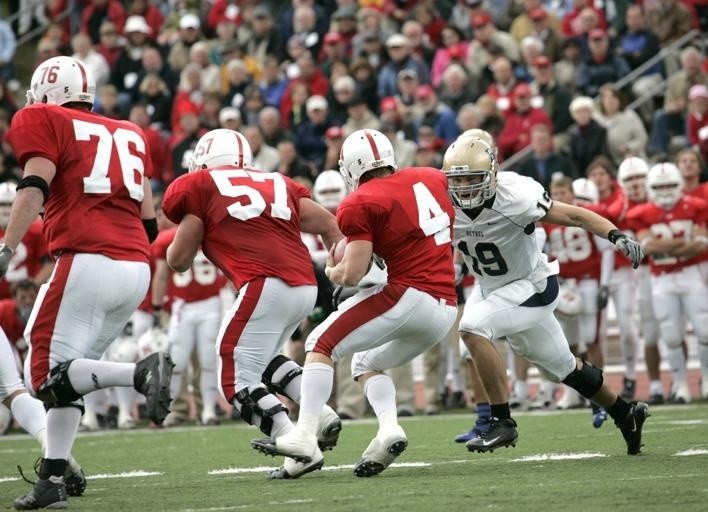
[54,451,88,498]
[454,413,492,443]
[614,400,651,456]
[466,416,520,453]
[133,347,175,424]
[76,404,219,429]
[591,400,606,428]
[395,375,708,417]
[10,473,67,512]
[250,403,408,479]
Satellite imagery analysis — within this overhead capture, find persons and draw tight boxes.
[1,1,708,429]
[1,55,174,511]
[443,128,650,455]
[162,128,347,480]
[250,129,458,477]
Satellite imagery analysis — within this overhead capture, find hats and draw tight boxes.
[123,1,623,123]
[687,82,708,101]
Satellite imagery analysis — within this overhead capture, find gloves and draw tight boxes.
[605,229,646,269]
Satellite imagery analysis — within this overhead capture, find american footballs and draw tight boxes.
[334,237,372,276]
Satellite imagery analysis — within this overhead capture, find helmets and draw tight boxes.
[0,183,21,228]
[190,127,252,174]
[336,126,397,187]
[24,52,99,110]
[440,124,498,210]
[314,168,346,212]
[572,156,684,204]
